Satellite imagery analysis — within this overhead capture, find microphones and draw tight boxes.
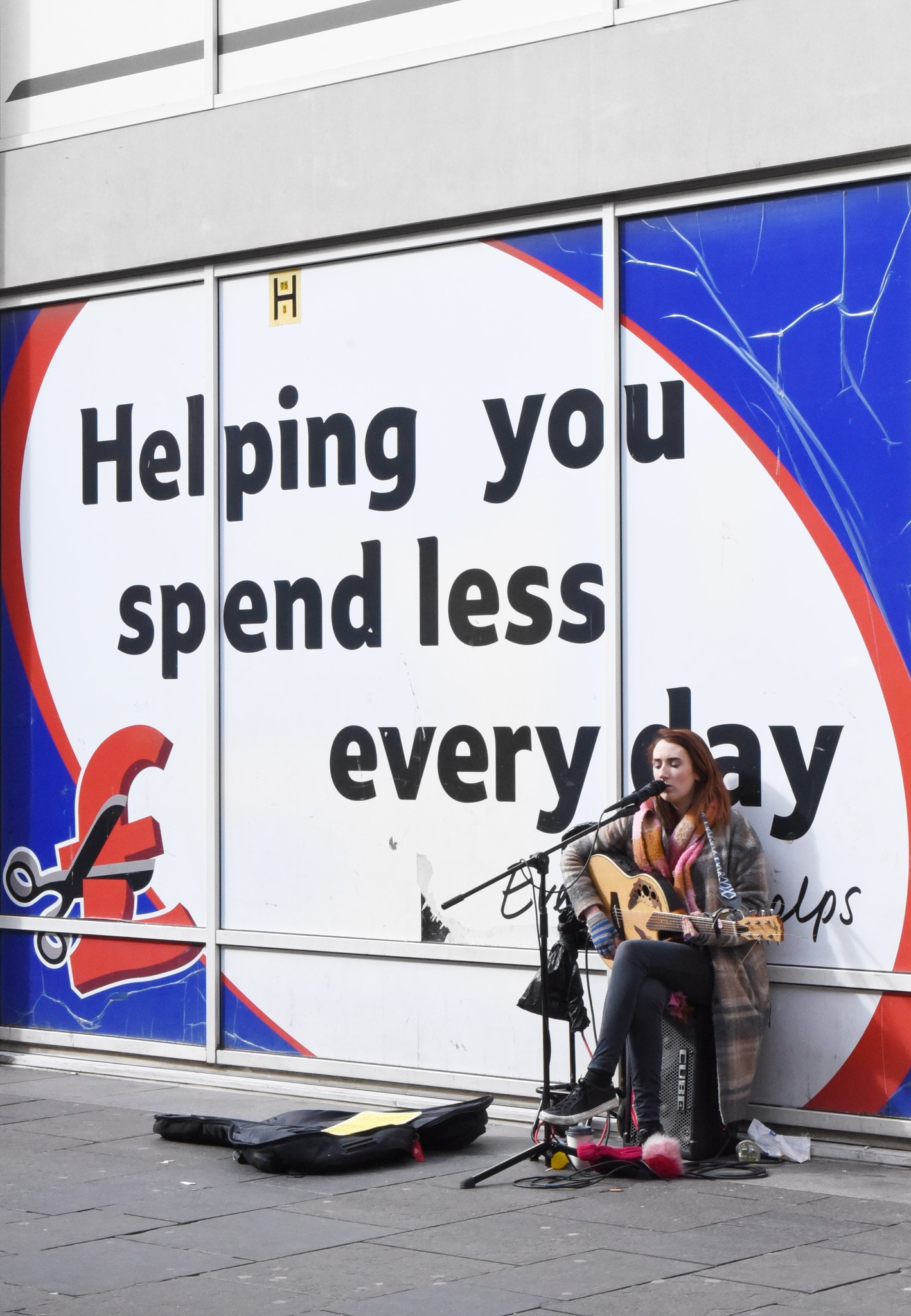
[604,780,665,812]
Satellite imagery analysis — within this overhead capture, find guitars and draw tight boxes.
[583,850,786,971]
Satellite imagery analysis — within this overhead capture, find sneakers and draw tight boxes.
[637,1126,667,1147]
[538,1080,620,1125]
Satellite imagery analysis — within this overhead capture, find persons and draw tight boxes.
[537,725,776,1157]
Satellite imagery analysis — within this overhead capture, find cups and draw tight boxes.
[565,1125,595,1169]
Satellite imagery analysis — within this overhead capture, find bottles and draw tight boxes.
[736,1139,760,1165]
